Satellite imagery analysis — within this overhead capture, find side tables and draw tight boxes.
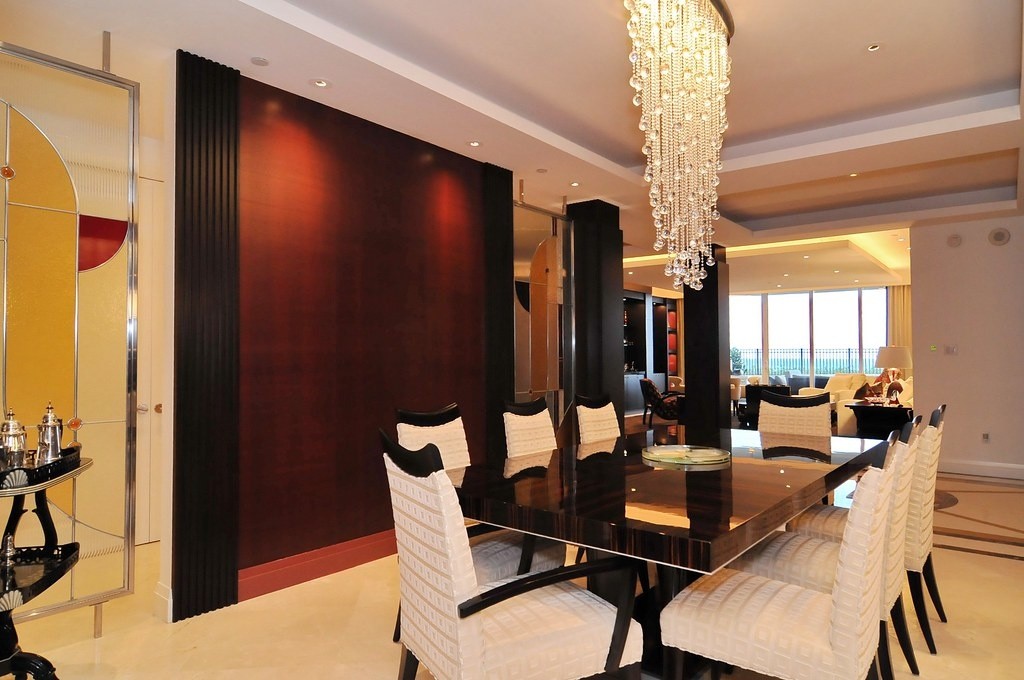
[843,403,912,442]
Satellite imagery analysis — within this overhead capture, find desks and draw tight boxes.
[446,424,885,680]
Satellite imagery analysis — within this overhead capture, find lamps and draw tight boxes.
[875,344,913,405]
[624,0,735,291]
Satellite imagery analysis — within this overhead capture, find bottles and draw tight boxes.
[34,400,62,469]
[0,405,25,470]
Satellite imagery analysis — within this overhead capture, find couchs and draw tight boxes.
[744,384,837,436]
[798,373,914,436]
[788,377,830,395]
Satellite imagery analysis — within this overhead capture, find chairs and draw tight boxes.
[740,376,762,398]
[769,376,784,386]
[785,369,801,384]
[639,378,685,429]
[378,390,947,680]
[668,375,685,393]
[730,377,741,415]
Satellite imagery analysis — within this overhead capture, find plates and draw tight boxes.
[641,458,730,471]
[642,444,730,464]
[865,397,890,402]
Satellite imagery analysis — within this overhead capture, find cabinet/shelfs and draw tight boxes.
[0,458,94,680]
[624,288,645,375]
